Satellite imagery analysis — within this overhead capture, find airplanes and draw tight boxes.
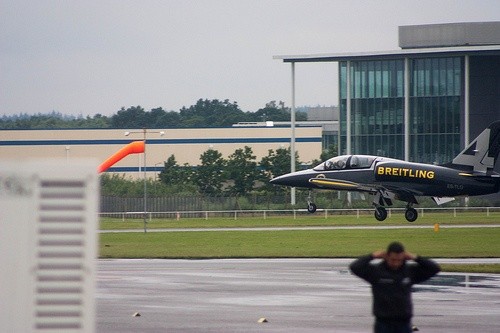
[269,121,500,222]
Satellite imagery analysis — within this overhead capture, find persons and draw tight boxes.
[349,242,441,333]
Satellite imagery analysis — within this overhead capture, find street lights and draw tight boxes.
[124,130,165,233]
